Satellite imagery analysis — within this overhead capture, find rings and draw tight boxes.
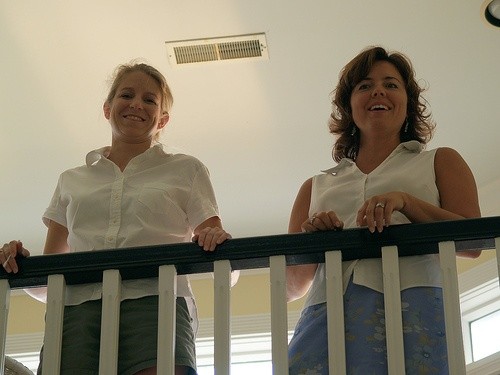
[0,250,3,252]
[311,217,315,225]
[376,203,385,208]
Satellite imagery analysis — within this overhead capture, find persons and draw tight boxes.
[287,48,483,375]
[0,63,232,375]
